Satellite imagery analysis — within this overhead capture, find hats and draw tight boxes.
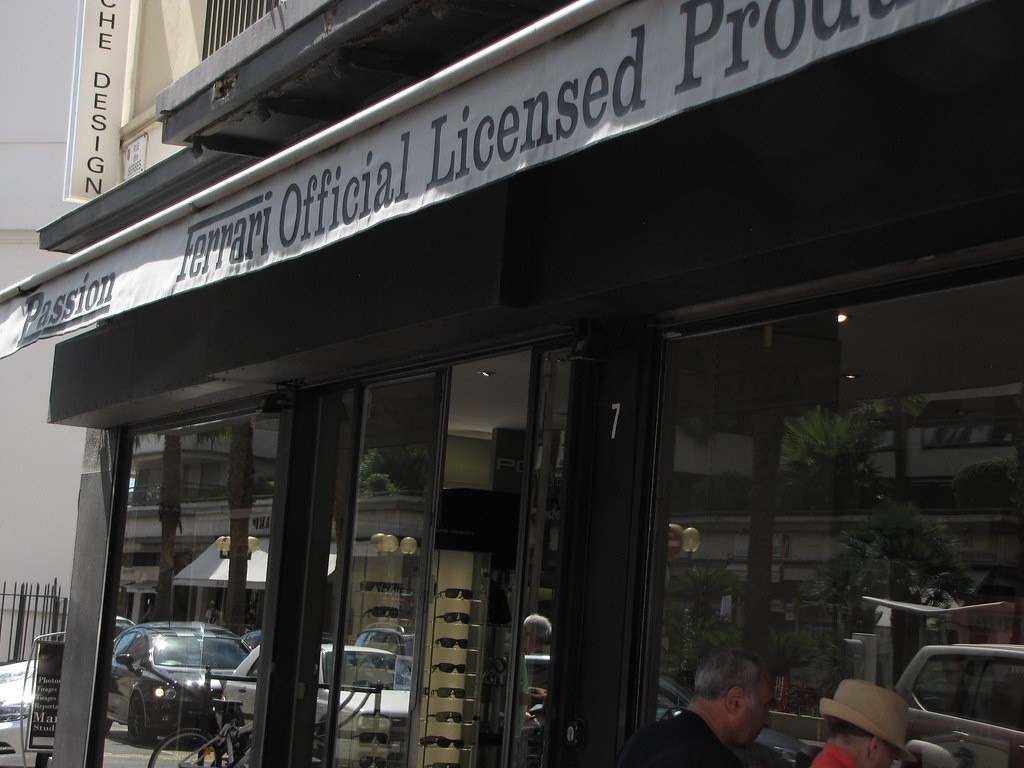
[819,677,915,764]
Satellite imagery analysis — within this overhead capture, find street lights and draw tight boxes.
[371,532,418,621]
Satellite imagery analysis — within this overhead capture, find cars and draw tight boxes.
[90,615,1024,768]
[0,658,63,768]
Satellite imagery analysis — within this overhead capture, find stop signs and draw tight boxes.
[666,527,683,560]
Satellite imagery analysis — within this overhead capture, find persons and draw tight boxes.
[512,613,552,768]
[613,646,777,768]
[204,600,220,624]
[809,678,918,768]
